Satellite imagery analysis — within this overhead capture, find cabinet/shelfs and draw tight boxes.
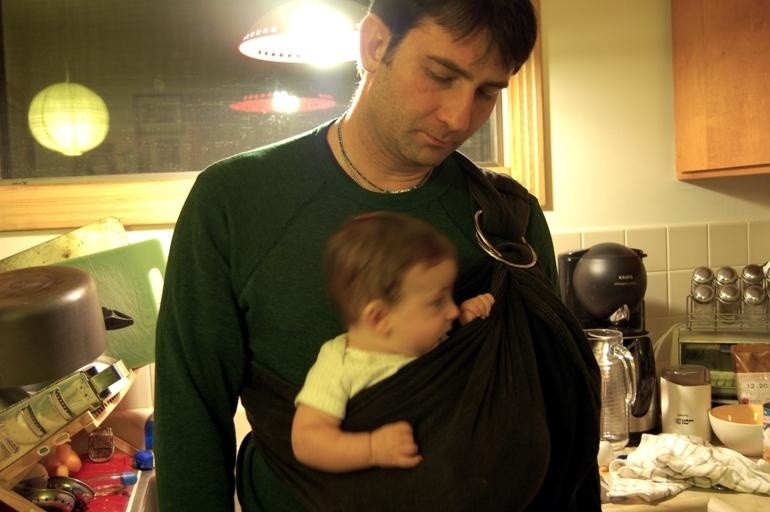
[670,0,770,182]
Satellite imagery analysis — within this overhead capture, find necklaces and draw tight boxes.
[338,111,432,193]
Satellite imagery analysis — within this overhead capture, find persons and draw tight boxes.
[291,211,495,466]
[152,0,601,512]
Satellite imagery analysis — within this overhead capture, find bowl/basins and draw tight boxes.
[709,404,765,457]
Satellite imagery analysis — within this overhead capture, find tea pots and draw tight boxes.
[579,328,638,451]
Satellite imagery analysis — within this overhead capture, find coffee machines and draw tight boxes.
[554,242,662,447]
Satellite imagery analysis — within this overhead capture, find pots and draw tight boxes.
[0,268,135,400]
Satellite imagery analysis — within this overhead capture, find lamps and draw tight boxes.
[26,0,111,157]
[229,62,336,116]
[237,0,362,66]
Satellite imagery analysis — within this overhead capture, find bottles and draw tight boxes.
[659,364,712,446]
[89,427,115,462]
[691,265,767,324]
[81,472,137,496]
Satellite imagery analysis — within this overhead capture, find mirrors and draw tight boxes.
[0,0,548,234]
